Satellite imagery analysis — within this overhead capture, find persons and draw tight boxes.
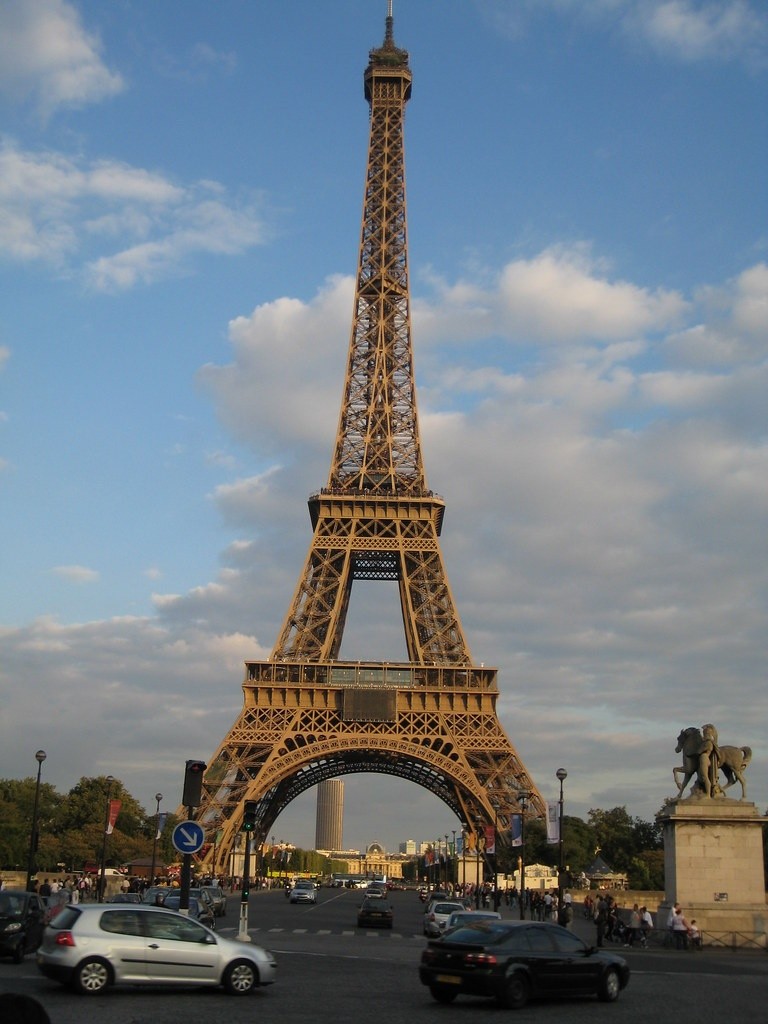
[150,891,172,909]
[439,880,573,932]
[39,865,182,910]
[685,723,720,800]
[194,872,290,891]
[582,892,703,950]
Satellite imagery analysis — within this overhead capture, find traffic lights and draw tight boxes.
[182,760,207,808]
[242,799,257,832]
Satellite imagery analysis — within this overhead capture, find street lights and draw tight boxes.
[433,823,467,892]
[98,775,114,903]
[556,768,567,927]
[26,750,46,891]
[211,813,288,885]
[476,815,481,909]
[516,793,532,920]
[493,804,500,913]
[150,793,162,886]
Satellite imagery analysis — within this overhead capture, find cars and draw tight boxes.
[107,884,228,931]
[0,890,50,964]
[36,903,278,997]
[290,882,318,905]
[354,878,402,929]
[417,883,502,938]
[419,920,631,1010]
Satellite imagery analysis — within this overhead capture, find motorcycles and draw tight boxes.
[284,885,290,897]
[419,892,429,903]
[317,883,321,891]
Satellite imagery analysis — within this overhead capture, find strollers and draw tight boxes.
[614,915,631,943]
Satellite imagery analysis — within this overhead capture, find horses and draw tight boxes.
[673,727,752,802]
